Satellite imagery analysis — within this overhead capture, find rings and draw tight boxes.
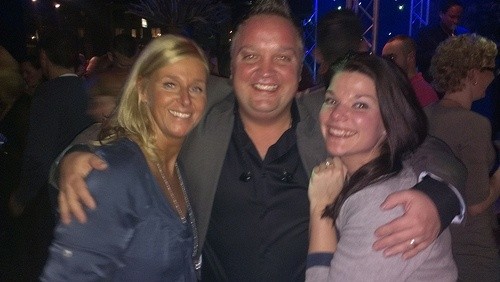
[325,160,332,167]
[410,238,416,248]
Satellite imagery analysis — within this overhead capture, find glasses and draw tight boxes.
[479,65,500,78]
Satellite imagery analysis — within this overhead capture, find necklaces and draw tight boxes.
[147,150,199,271]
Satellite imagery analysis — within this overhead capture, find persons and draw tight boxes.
[0,0,500,282]
